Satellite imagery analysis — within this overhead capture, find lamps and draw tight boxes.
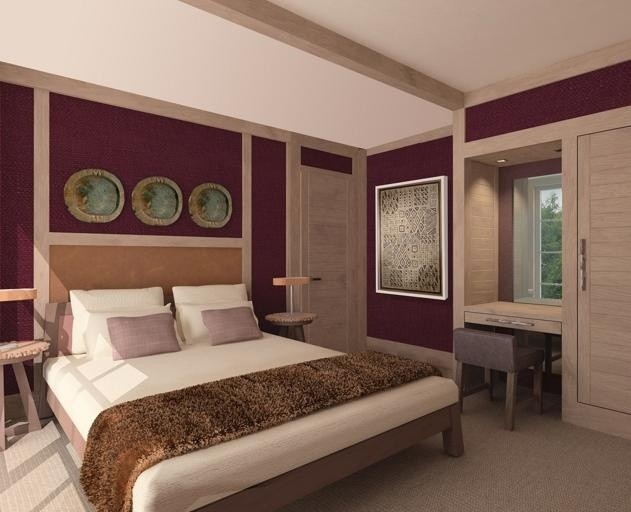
[0,288,37,353]
[273,277,310,315]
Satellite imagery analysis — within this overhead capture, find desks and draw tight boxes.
[0,341,51,451]
[265,313,313,342]
[464,302,562,335]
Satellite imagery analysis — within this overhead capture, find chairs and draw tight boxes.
[454,328,544,431]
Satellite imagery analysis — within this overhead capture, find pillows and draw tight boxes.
[70,283,262,361]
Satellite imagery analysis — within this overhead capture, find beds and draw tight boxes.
[39,303,464,512]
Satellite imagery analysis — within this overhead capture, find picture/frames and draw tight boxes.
[375,175,448,301]
